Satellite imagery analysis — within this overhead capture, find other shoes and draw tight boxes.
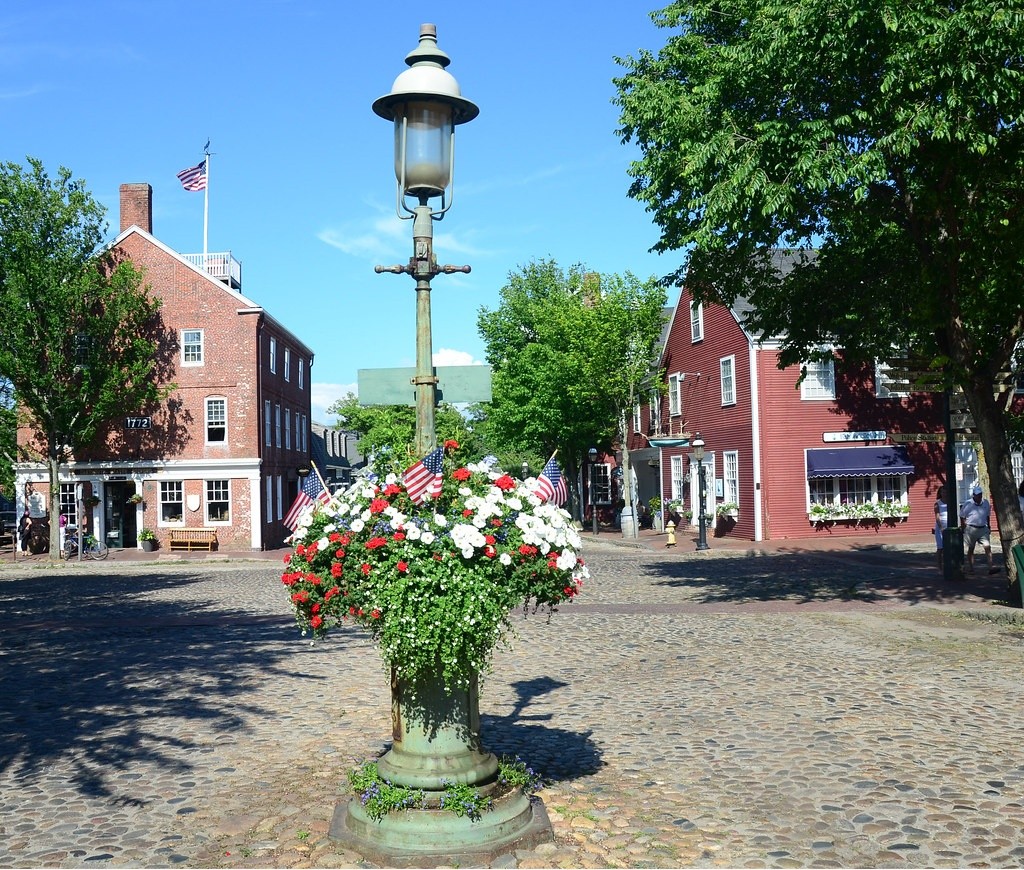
[22,552,33,556]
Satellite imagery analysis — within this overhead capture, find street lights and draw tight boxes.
[521,462,528,480]
[691,434,711,550]
[587,448,599,535]
[345,23,533,851]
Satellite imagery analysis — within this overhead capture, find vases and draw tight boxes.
[132,500,142,504]
[92,501,98,506]
[376,657,498,789]
[143,538,154,552]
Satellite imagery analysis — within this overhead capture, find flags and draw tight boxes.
[176,159,207,194]
[529,455,569,508]
[400,445,446,504]
[282,468,332,535]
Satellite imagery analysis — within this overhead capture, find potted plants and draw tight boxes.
[136,528,155,552]
[80,496,102,507]
[663,498,683,512]
[808,499,911,521]
[649,496,662,518]
[125,494,143,504]
[715,502,738,517]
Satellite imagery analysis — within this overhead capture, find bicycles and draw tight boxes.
[29,530,50,554]
[64,534,108,561]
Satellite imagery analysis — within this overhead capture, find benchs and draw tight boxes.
[168,527,217,552]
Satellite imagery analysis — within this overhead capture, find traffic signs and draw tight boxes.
[881,356,1016,443]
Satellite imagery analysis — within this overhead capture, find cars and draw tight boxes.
[0,511,16,530]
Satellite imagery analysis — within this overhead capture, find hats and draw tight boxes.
[24,511,29,516]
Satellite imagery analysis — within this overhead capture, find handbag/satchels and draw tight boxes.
[19,525,24,533]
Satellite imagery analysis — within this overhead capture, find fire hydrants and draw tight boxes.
[664,520,677,545]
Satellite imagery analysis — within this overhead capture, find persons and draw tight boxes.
[48,509,68,558]
[932,480,1024,578]
[17,511,32,557]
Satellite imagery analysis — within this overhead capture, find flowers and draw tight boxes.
[280,441,591,695]
